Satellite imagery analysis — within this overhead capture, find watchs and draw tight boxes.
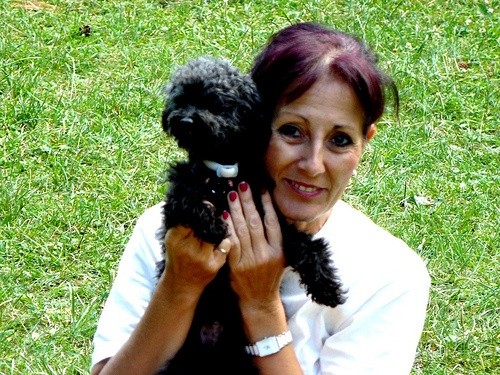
[246,331,292,357]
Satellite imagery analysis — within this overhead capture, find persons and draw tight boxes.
[88,19,429,375]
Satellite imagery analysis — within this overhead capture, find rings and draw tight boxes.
[217,247,230,254]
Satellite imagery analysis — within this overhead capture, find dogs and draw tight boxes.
[151,56,347,375]
